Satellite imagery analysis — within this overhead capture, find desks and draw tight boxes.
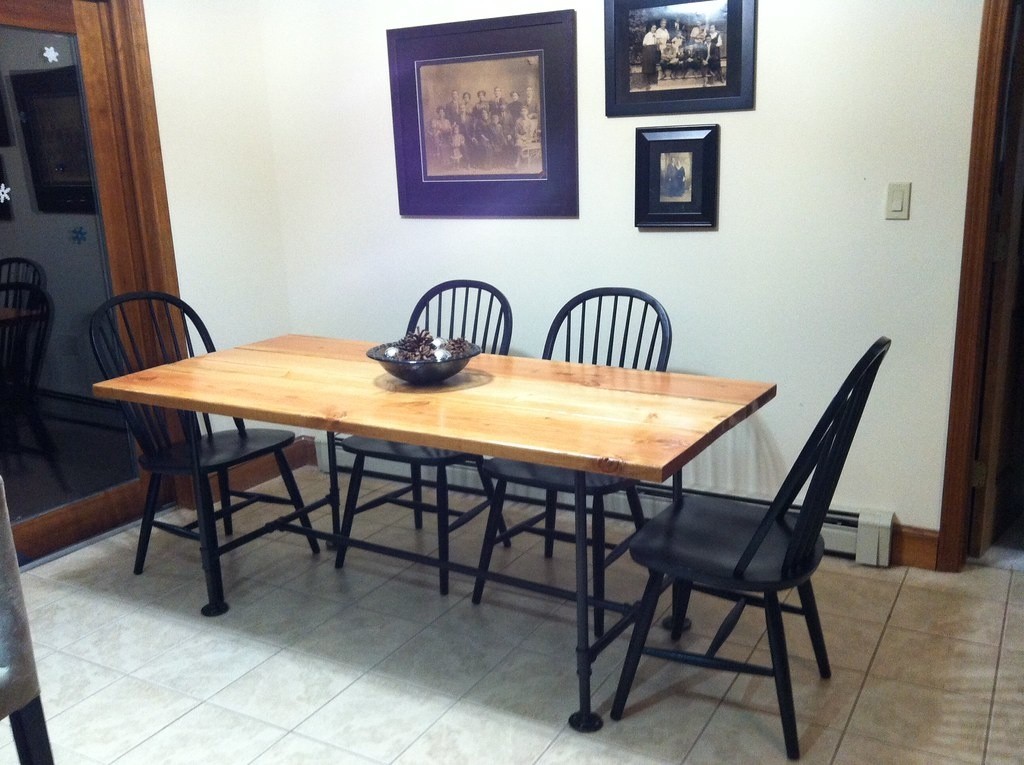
[92,335,774,732]
[0,307,42,326]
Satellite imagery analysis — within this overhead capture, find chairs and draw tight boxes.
[611,336,891,760]
[0,257,74,495]
[336,280,513,596]
[471,285,673,637]
[90,290,319,582]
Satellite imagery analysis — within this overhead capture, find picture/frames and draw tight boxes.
[604,0,756,117]
[635,123,718,227]
[386,8,580,218]
[8,65,97,216]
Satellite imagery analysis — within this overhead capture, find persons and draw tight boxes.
[667,157,686,197]
[642,18,724,85]
[426,85,541,171]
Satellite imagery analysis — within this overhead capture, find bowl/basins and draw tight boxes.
[366,341,482,385]
